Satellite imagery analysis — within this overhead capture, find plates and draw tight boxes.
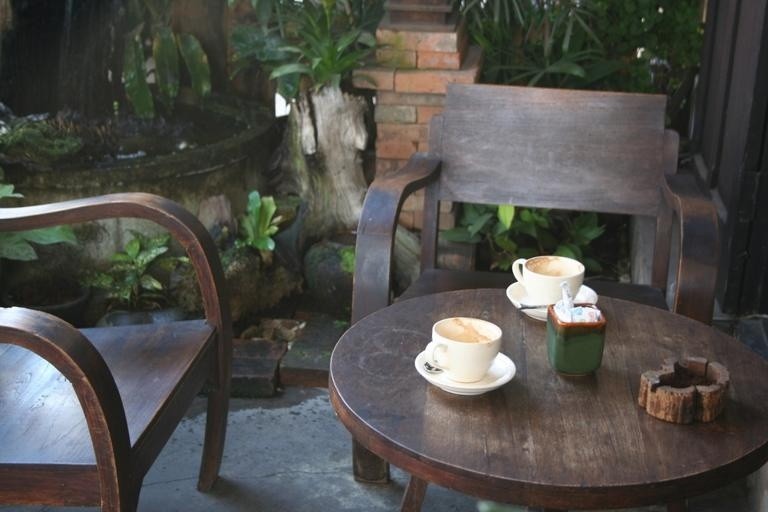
[414,351,517,396]
[505,282,598,321]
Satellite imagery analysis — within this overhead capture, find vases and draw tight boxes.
[151,306,188,324]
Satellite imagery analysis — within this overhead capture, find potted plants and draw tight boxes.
[0,0,279,280]
[7,219,110,327]
[93,229,171,326]
[441,202,607,281]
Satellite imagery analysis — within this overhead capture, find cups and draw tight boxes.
[426,318,503,382]
[545,304,605,379]
[511,254,585,310]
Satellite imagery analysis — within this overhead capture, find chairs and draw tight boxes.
[1,193,233,512]
[351,81,720,483]
